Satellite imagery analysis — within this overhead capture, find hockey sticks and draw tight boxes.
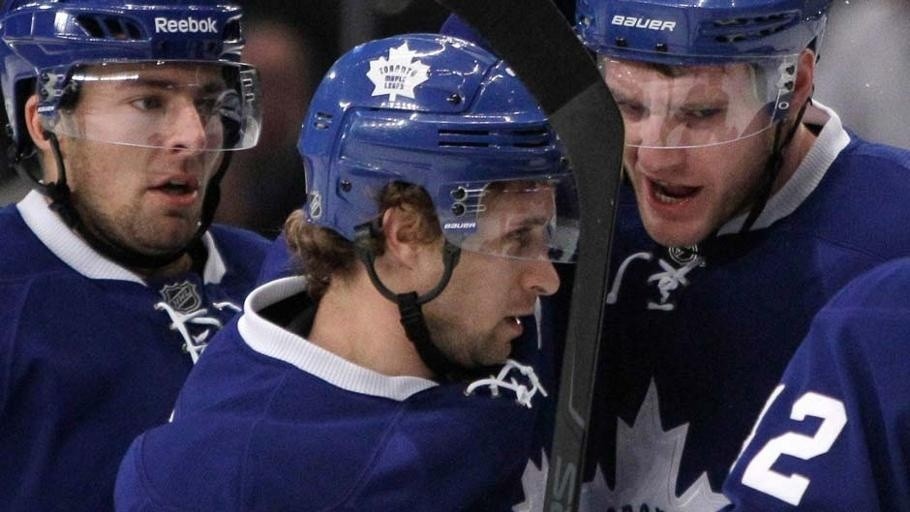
[448,1,624,512]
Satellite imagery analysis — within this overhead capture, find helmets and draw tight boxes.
[297,33,582,263]
[0,0,263,159]
[572,1,827,148]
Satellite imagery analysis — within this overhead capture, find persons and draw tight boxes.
[532,0,908,512]
[722,257,910,512]
[114,35,580,512]
[0,1,272,512]
